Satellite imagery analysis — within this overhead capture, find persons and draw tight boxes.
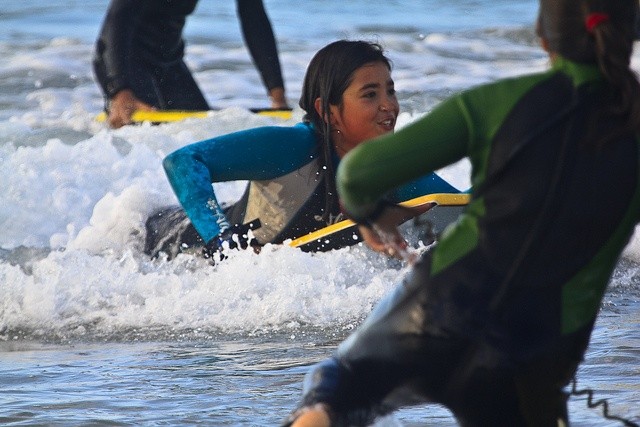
[278,0,640,426]
[132,39,464,264]
[91,0,289,129]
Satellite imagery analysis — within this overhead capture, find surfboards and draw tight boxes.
[269,193,470,252]
[95,107,294,127]
